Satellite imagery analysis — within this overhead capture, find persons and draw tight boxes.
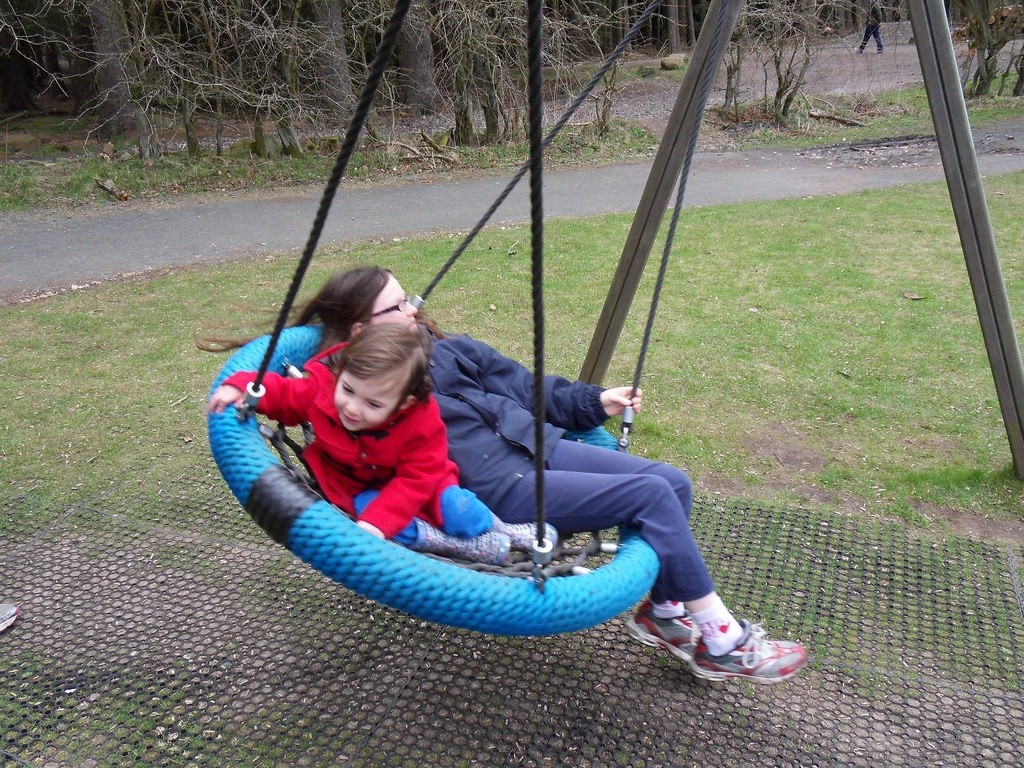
[207,324,557,566]
[853,0,884,54]
[197,263,807,684]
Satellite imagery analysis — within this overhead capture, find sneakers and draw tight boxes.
[622,604,702,666]
[691,619,809,686]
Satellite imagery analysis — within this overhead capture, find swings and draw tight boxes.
[207,1,728,637]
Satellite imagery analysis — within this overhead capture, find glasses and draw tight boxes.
[369,294,423,321]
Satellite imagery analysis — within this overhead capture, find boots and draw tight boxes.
[410,517,511,565]
[490,513,559,554]
